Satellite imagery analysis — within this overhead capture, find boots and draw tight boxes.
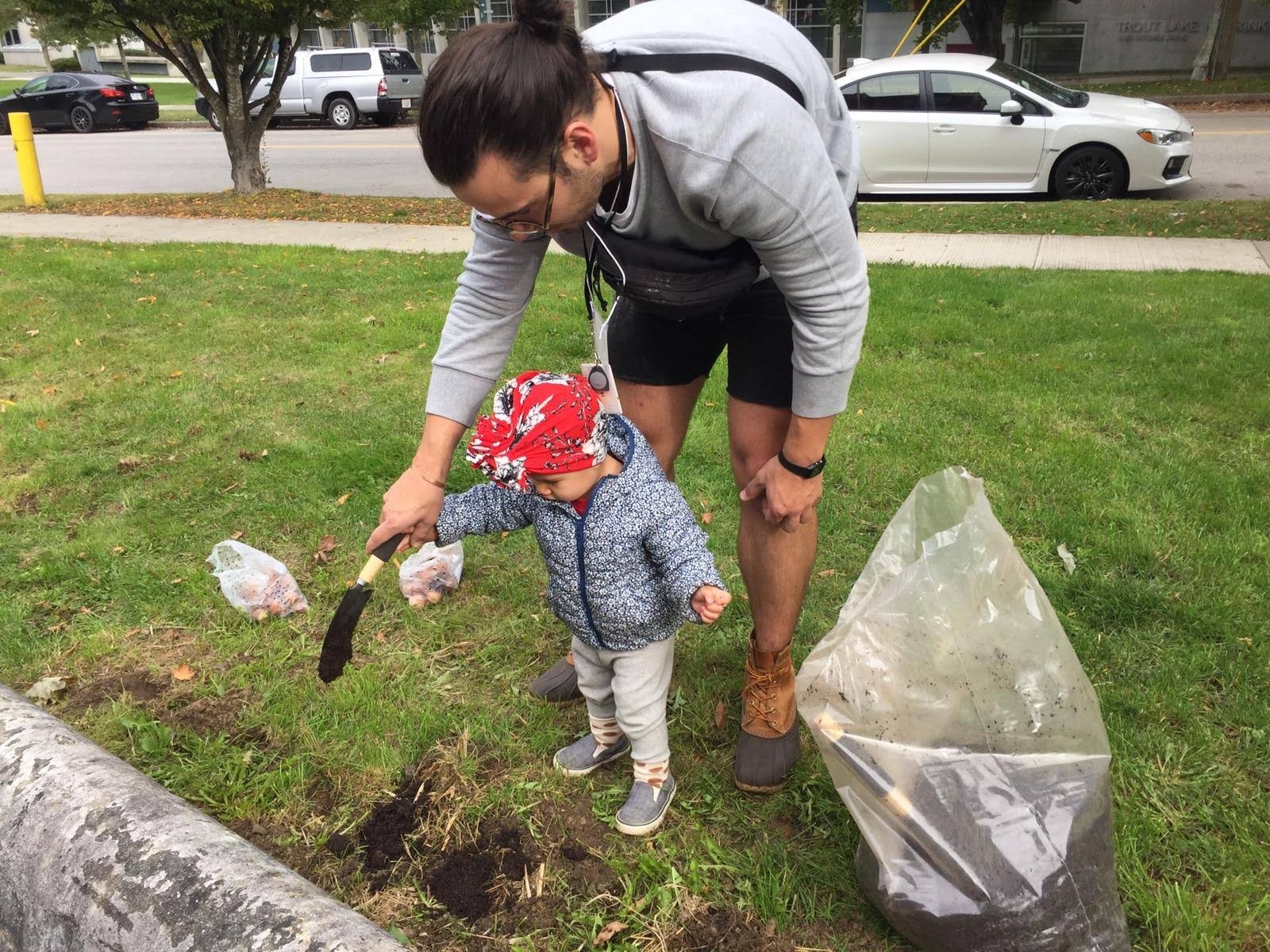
[526,648,588,707]
[734,625,801,794]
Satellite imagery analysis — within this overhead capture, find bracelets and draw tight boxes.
[410,463,447,489]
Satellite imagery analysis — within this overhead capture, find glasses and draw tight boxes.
[474,132,566,237]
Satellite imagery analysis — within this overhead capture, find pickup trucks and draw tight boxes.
[195,47,426,132]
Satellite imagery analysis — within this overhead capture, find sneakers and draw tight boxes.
[553,730,631,775]
[613,768,677,836]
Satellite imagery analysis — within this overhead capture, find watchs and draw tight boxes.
[777,442,827,480]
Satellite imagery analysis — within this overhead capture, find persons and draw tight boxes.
[365,0,869,795]
[394,370,731,836]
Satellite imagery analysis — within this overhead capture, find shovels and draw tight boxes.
[319,534,406,682]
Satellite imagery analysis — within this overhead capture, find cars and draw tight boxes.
[0,72,159,134]
[832,53,1195,201]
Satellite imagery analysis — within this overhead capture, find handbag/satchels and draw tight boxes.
[398,538,464,606]
[206,539,309,622]
[795,458,1134,952]
[590,225,762,322]
